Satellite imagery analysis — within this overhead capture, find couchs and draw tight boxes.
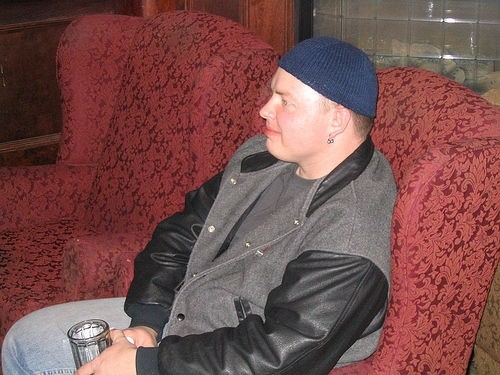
[0,8,499,375]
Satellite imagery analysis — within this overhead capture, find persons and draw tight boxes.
[0,35,397,375]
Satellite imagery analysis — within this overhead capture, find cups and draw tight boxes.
[67,319,112,370]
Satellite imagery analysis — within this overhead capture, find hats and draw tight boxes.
[279,36,377,118]
